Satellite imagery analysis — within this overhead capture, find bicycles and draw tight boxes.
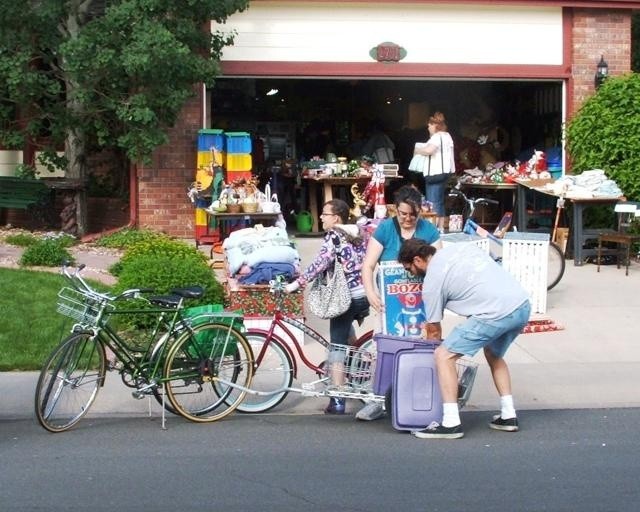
[41,261,240,414]
[212,279,375,413]
[448,189,566,290]
[36,264,255,432]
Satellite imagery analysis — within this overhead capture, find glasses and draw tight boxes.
[322,212,335,217]
[396,207,420,220]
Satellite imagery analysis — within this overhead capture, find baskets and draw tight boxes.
[55,286,117,328]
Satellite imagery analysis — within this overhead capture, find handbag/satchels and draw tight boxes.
[308,261,351,320]
[427,173,448,185]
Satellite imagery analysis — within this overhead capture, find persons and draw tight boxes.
[305,118,408,167]
[398,238,531,439]
[415,112,456,234]
[356,186,443,420]
[284,200,370,416]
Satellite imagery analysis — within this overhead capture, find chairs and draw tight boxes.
[596,205,636,275]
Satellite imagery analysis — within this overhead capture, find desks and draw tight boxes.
[517,176,625,267]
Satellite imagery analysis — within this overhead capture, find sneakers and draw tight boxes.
[488,415,519,432]
[355,400,386,420]
[324,397,346,415]
[413,420,464,439]
